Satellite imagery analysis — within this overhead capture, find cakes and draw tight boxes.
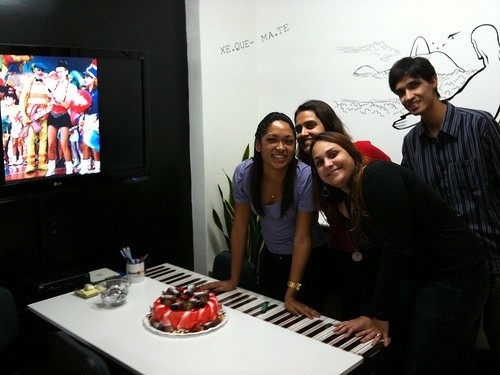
[148,283,223,334]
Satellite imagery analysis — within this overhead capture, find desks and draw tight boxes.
[27,261,391,375]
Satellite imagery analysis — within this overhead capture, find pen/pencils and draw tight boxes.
[120,247,148,264]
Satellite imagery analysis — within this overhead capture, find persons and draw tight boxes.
[309,131,490,375]
[295,100,392,254]
[197,112,321,320]
[388,56,500,375]
[0,54,101,182]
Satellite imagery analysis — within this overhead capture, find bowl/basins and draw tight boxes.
[96,279,131,306]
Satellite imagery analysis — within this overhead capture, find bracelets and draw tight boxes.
[287,280,302,290]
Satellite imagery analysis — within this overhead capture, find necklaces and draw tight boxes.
[54,80,69,104]
[272,194,276,198]
[346,215,363,262]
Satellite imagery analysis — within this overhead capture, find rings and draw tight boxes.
[376,333,383,336]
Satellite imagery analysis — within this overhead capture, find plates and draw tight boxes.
[143,311,229,335]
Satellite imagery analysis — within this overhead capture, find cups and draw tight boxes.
[126,259,145,283]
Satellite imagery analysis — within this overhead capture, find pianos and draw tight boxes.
[28,263,383,375]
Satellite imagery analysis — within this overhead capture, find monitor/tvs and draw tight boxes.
[0,42,155,201]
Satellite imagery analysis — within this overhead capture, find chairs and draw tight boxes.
[52,331,109,375]
[39,273,90,299]
